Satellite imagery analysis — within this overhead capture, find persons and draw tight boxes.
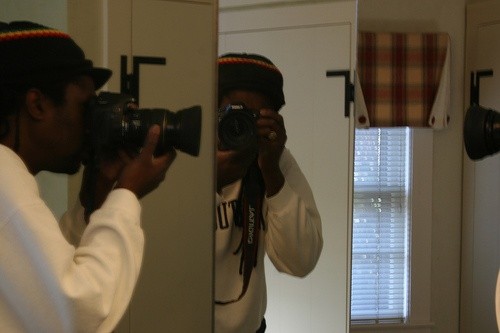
[0,19,177,333]
[213,52,323,333]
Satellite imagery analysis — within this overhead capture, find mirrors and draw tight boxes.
[212,0,500,333]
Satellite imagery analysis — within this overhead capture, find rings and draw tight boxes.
[269,130,276,139]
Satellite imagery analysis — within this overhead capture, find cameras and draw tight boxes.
[465,106,500,161]
[87,92,202,157]
[216,103,266,149]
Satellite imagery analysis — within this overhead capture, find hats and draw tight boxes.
[217,49,289,105]
[0,19,112,92]
